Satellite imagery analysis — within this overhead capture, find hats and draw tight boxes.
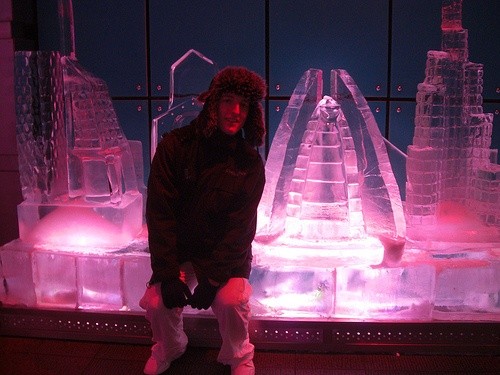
[195,66,268,152]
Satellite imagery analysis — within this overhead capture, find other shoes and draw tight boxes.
[231,360,255,375]
[144,344,186,375]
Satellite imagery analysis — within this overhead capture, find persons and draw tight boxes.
[139,66,268,375]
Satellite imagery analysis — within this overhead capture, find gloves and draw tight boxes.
[185,284,216,310]
[161,277,192,309]
